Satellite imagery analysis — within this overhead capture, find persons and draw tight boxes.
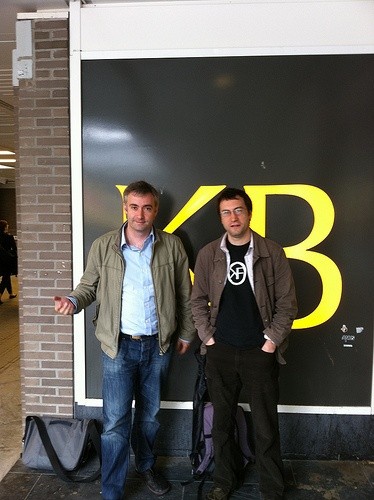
[54,180,197,500]
[191,188,298,500]
[0,219,18,304]
